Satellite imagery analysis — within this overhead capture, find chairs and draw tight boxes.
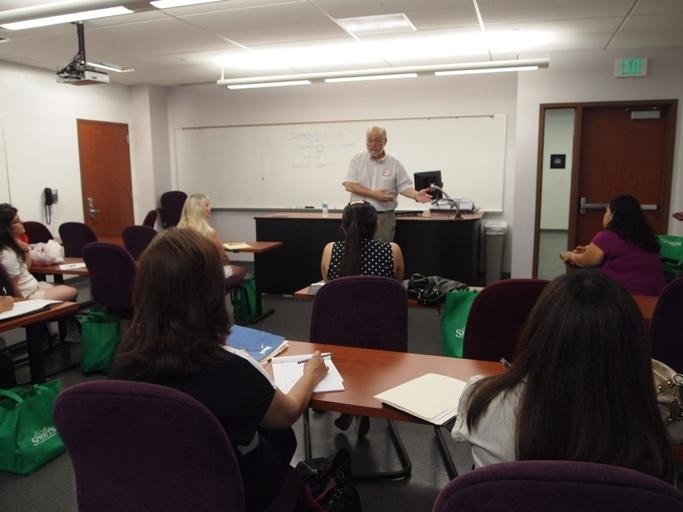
[52,378,339,512]
[300,274,414,484]
[19,220,56,245]
[161,190,188,228]
[431,277,558,483]
[80,240,138,320]
[120,223,160,258]
[426,458,682,512]
[58,221,97,260]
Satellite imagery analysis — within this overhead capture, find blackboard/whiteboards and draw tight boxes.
[172,113,506,214]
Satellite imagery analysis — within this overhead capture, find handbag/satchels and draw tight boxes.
[1,378,69,476]
[229,272,270,325]
[78,308,119,373]
[439,290,481,358]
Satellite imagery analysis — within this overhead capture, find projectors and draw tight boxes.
[56,55,110,85]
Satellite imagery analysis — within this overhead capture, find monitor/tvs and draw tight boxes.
[414,171,442,202]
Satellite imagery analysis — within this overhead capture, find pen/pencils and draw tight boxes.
[297,353,334,362]
[2,286,8,296]
[500,357,512,369]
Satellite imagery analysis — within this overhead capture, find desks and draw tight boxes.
[250,209,489,305]
[26,256,140,277]
[0,296,78,385]
[293,277,660,326]
[217,238,283,325]
[258,338,513,428]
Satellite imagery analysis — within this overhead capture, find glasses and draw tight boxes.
[10,218,20,225]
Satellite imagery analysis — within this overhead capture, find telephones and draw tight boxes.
[44,188,58,225]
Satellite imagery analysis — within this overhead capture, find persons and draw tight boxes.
[111,225,330,512]
[0,202,79,302]
[319,200,405,287]
[451,267,677,484]
[341,124,433,241]
[174,193,247,294]
[558,193,669,299]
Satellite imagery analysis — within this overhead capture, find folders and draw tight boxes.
[225,323,285,363]
